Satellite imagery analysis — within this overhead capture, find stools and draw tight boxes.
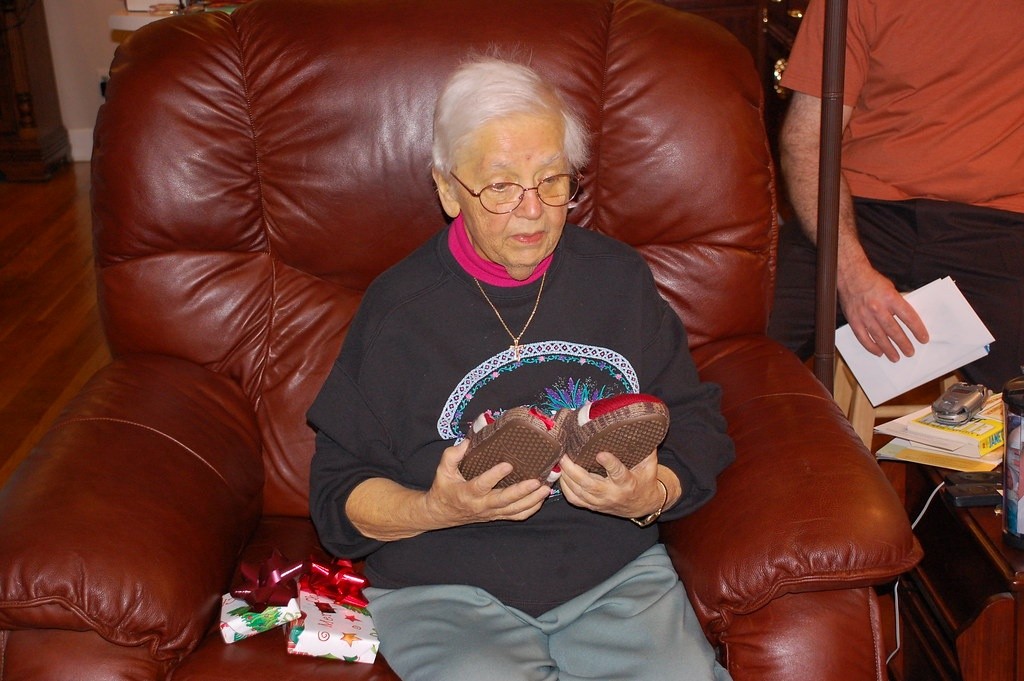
[832,291,966,452]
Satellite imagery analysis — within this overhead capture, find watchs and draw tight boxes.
[630,478,668,527]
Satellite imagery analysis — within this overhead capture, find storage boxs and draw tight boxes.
[283,586,381,663]
[220,588,302,643]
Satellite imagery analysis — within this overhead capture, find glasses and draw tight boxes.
[450,171,585,214]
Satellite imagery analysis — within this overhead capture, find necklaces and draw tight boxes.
[474,274,546,360]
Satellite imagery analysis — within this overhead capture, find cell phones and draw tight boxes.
[932,381,987,424]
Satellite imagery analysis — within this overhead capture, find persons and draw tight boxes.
[775,0,1024,413]
[305,57,735,681]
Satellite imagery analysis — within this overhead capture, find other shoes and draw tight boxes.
[456,393,568,495]
[556,394,671,489]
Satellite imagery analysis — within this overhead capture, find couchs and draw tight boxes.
[0,0,925,681]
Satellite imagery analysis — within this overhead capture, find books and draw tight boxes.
[873,392,1008,473]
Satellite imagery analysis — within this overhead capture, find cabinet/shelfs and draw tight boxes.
[898,461,1024,681]
